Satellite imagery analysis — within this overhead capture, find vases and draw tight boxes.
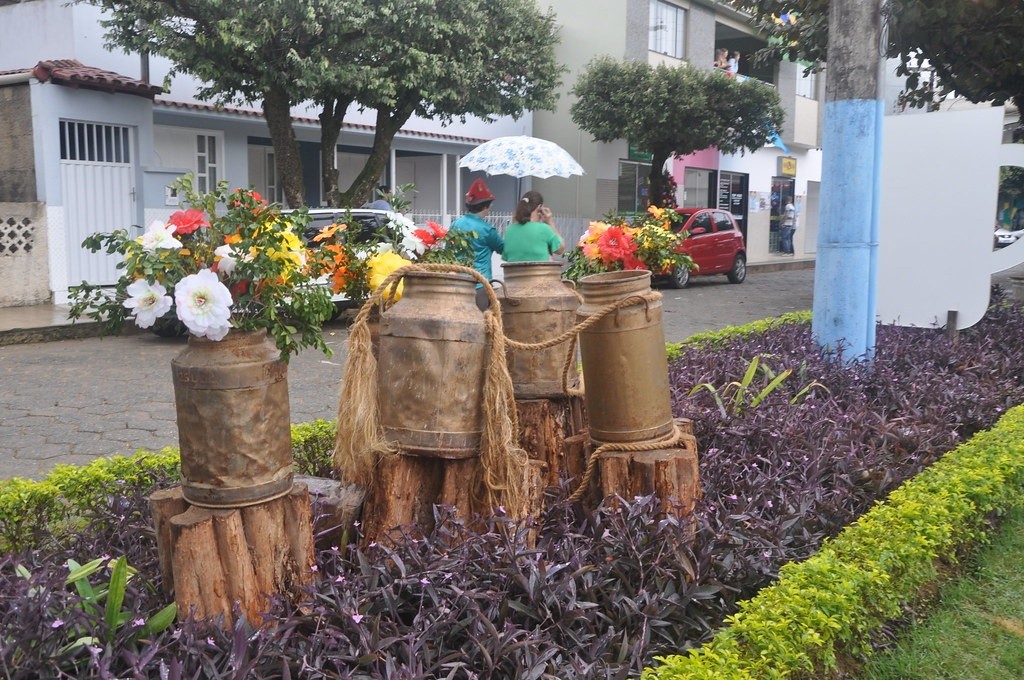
[489,260,581,395]
[376,272,487,460]
[169,324,302,508]
[578,268,676,443]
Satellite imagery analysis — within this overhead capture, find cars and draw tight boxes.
[994,226,1024,246]
[637,207,747,289]
[150,208,426,338]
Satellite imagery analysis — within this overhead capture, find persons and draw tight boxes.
[367,186,393,210]
[778,195,796,257]
[501,190,565,262]
[443,176,519,311]
[714,48,741,74]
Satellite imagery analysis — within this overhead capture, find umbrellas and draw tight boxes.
[456,135,587,202]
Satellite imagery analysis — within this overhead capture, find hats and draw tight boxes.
[464,176,497,205]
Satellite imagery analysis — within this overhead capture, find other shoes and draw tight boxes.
[782,253,794,257]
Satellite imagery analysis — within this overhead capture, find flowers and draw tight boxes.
[558,204,700,281]
[313,179,478,303]
[64,170,338,366]
[410,221,481,275]
